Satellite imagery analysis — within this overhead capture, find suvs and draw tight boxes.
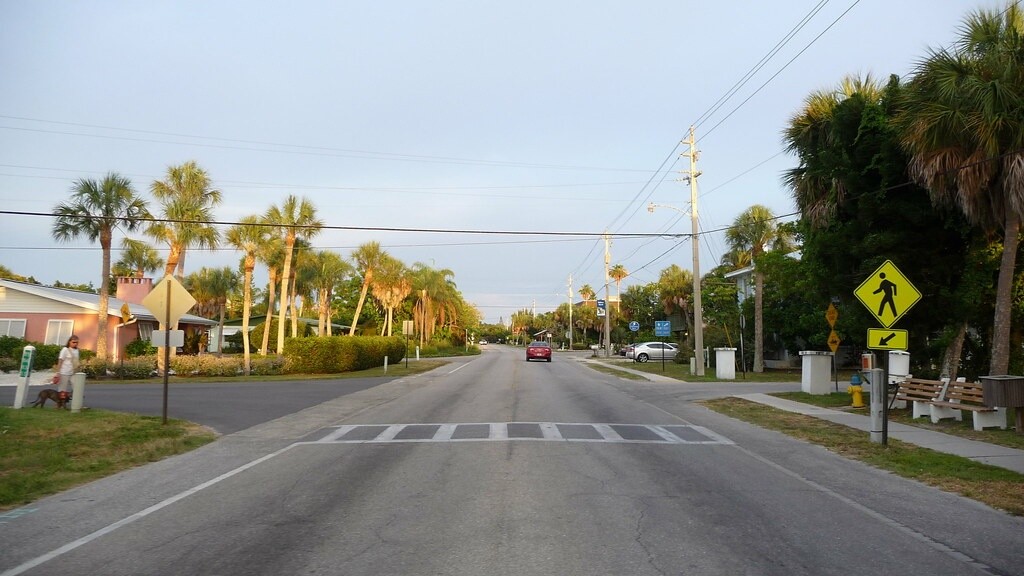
[525,340,552,362]
[496,339,504,344]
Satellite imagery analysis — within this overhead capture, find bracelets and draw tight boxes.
[57,372,61,374]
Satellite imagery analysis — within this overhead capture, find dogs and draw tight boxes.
[30,389,72,411]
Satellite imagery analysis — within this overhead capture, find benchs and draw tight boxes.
[895,376,1007,431]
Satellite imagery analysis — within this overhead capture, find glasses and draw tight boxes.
[71,340,78,343]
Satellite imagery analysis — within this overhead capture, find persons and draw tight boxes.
[56,335,81,411]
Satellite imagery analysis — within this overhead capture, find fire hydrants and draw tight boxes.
[847,374,866,407]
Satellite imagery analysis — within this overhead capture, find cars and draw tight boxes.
[619,343,640,356]
[627,342,680,363]
[480,339,488,345]
